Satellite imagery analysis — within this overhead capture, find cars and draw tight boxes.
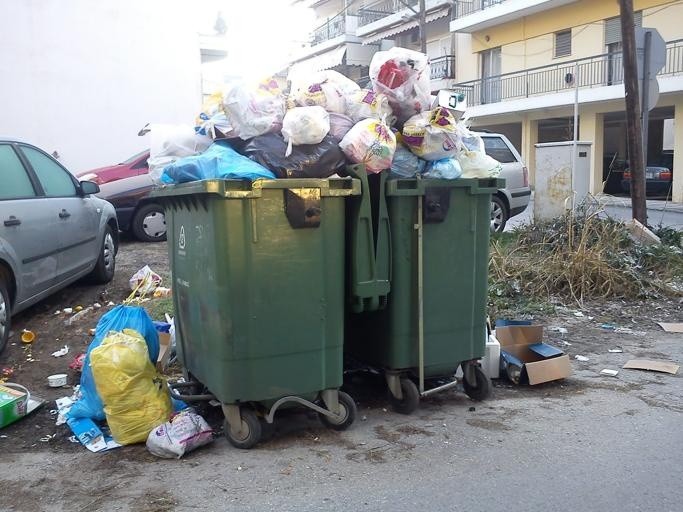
[0,135,119,355]
[603,155,671,197]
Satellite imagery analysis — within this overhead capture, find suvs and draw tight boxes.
[459,129,532,232]
[72,134,211,245]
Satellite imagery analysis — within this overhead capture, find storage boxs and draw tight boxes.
[493,319,577,390]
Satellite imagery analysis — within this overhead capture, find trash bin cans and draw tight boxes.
[152,165,376,451]
[345,169,508,415]
